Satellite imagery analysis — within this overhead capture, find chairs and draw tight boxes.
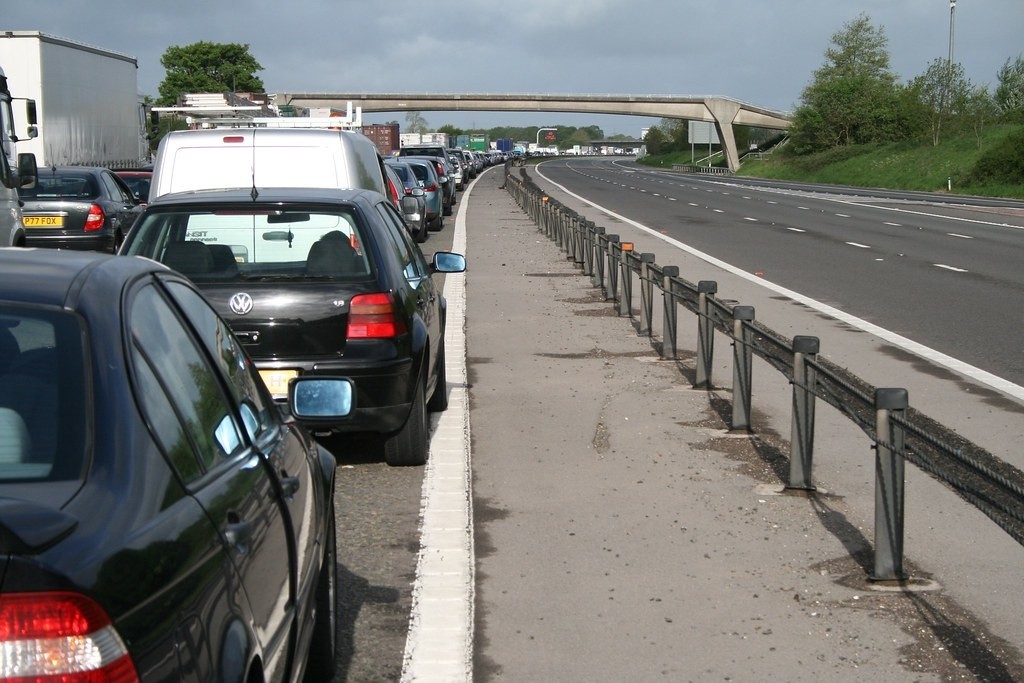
[159,240,364,281]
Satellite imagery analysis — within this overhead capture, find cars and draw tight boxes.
[379,143,641,241]
[109,170,152,208]
[0,241,356,679]
[115,130,467,468]
[13,164,141,255]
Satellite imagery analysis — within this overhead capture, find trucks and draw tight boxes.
[1,29,161,168]
[1,64,39,249]
